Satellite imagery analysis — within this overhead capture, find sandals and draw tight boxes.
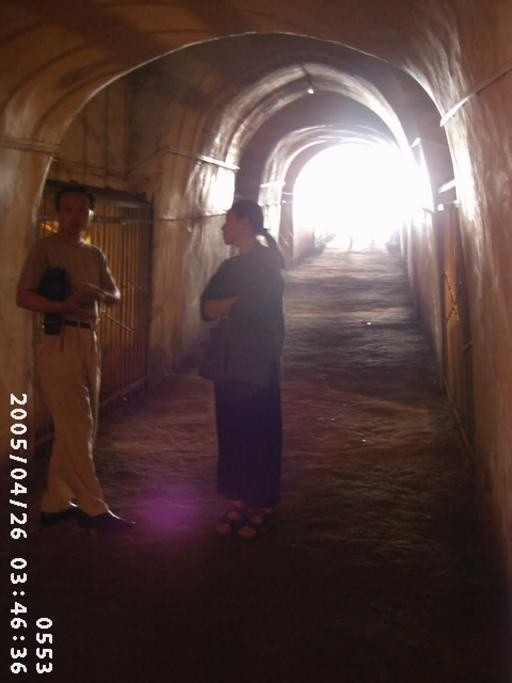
[213,503,245,537]
[235,503,282,540]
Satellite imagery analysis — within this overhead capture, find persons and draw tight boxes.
[12,178,137,532]
[198,199,286,542]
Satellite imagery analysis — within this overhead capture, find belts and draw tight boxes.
[63,320,90,330]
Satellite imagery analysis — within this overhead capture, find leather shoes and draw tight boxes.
[40,503,80,526]
[77,511,137,530]
